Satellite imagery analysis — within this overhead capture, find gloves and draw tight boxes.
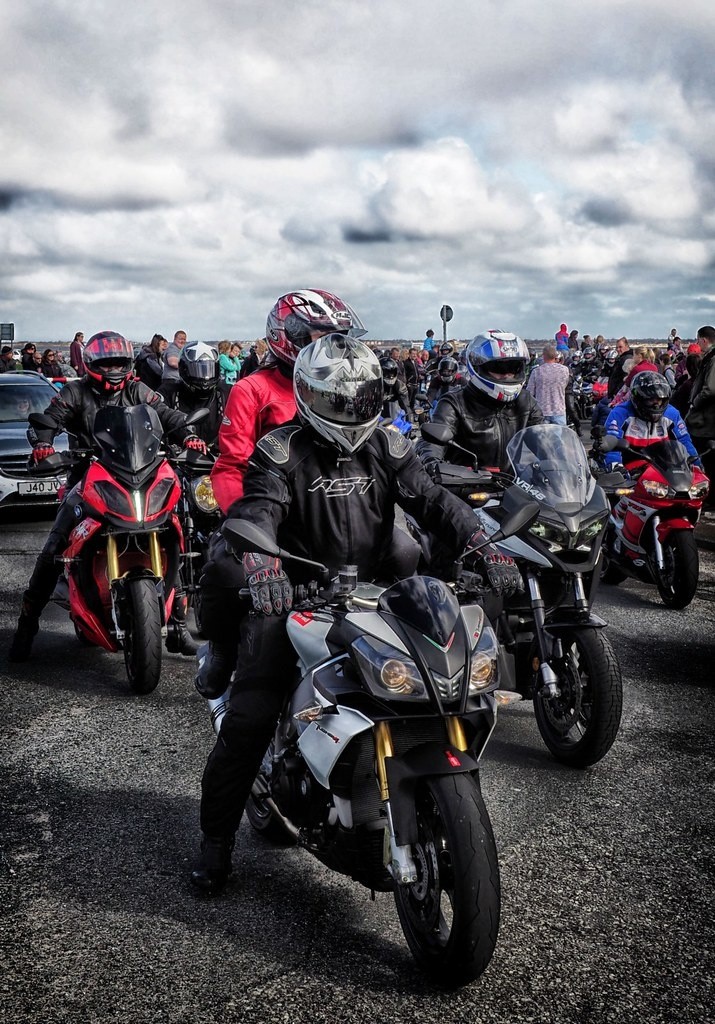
[182,435,207,455]
[242,555,295,615]
[32,442,54,465]
[465,535,526,600]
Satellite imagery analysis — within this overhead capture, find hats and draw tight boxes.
[2,346,13,354]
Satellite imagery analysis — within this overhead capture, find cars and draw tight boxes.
[12,349,23,364]
[0,369,74,508]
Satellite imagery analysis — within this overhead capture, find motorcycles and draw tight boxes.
[587,434,715,611]
[29,407,210,698]
[377,361,467,448]
[165,440,225,595]
[197,498,547,993]
[418,421,631,775]
[561,359,612,439]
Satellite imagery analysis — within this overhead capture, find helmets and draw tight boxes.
[466,329,529,404]
[438,358,459,382]
[380,357,399,385]
[83,332,136,394]
[630,371,672,424]
[293,333,385,454]
[179,341,221,394]
[266,288,353,377]
[439,342,454,358]
[571,343,618,367]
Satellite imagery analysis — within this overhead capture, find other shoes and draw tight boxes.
[192,832,235,894]
[576,427,584,437]
[196,643,237,699]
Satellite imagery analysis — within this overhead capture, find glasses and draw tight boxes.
[48,354,55,357]
[37,358,42,360]
[29,348,35,351]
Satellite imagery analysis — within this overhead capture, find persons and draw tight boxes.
[0,331,275,407]
[190,289,524,896]
[363,324,715,525]
[7,332,209,673]
[11,392,35,420]
[414,329,570,583]
[154,342,231,446]
[599,371,705,555]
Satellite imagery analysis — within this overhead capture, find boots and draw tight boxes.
[165,591,199,656]
[9,591,49,663]
